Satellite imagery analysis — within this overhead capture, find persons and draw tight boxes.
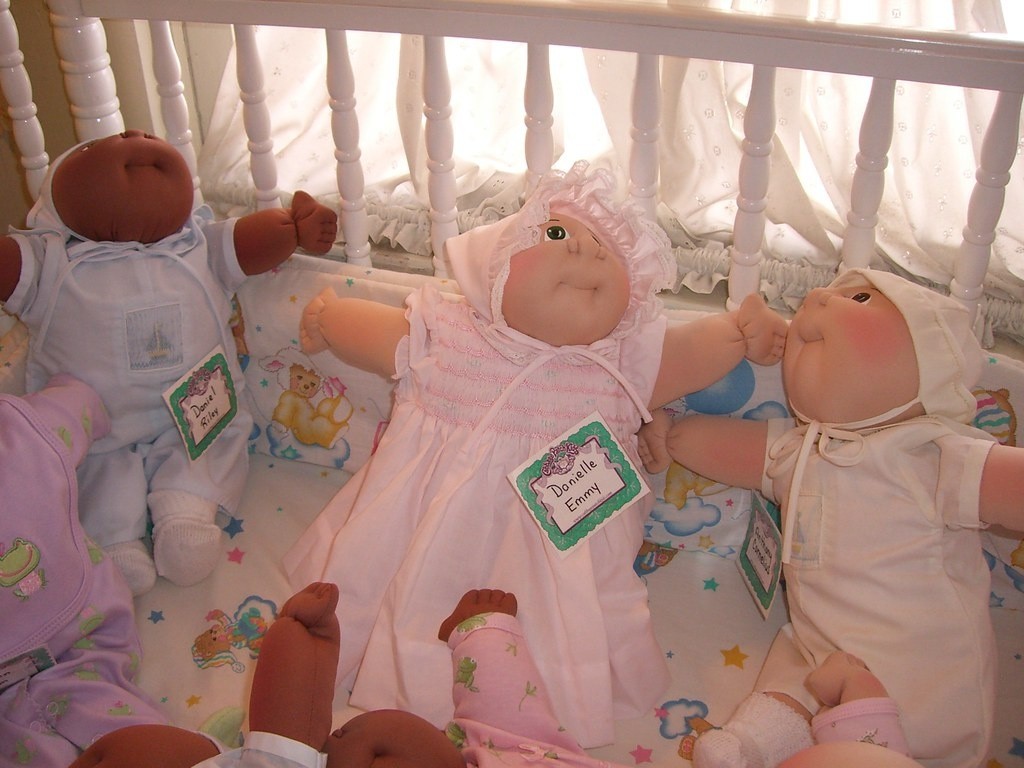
[632,268,1024,768]
[0,128,1024,768]
